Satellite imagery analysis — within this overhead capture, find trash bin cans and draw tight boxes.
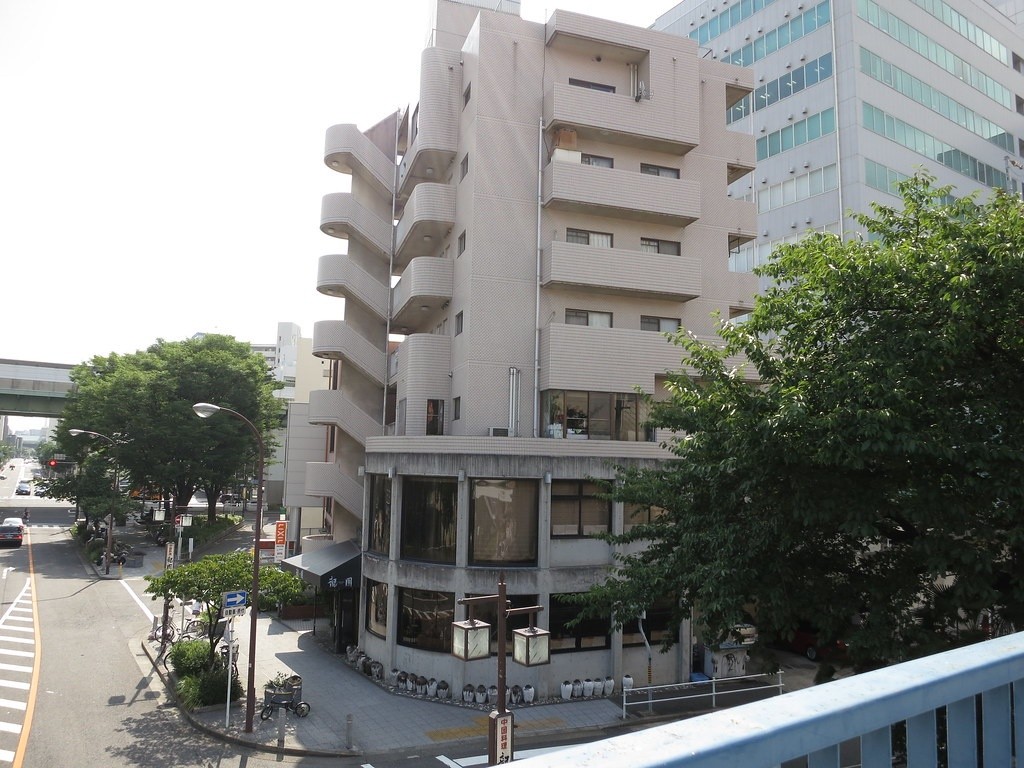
[285,675,303,707]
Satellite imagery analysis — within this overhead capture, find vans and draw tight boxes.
[0,524,22,547]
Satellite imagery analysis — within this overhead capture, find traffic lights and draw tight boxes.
[50,459,56,468]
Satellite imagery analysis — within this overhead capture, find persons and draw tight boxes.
[150,507,154,519]
[23,507,30,521]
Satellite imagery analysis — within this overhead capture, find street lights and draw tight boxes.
[451,571,552,766]
[193,402,264,735]
[151,495,193,642]
[70,429,118,574]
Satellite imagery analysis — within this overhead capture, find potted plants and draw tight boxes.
[567,406,586,429]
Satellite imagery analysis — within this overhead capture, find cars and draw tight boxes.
[34,473,50,495]
[783,625,848,661]
[17,480,30,494]
[0,474,7,480]
[3,518,24,532]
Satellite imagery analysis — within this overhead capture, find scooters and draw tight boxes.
[96,539,134,566]
[145,523,166,547]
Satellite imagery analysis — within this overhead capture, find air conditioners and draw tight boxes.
[487,427,515,437]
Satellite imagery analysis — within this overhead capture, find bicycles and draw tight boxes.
[164,651,175,671]
[221,639,239,680]
[154,609,205,643]
[260,682,310,720]
[157,628,191,657]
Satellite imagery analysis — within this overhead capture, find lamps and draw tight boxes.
[328,227,335,234]
[332,161,339,166]
[423,235,432,241]
[401,327,408,332]
[358,466,365,476]
[545,471,552,485]
[421,306,430,311]
[327,289,335,295]
[426,167,433,174]
[458,469,465,482]
[388,466,396,479]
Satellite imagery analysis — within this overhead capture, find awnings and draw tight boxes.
[281,539,362,589]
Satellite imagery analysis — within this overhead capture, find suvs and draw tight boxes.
[219,494,243,504]
